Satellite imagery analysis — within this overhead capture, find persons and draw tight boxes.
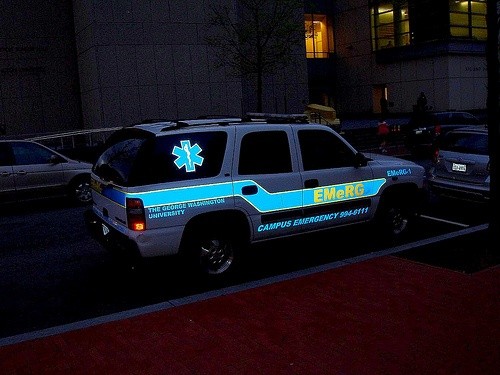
[380,92,391,118]
[417,91,427,104]
[377,115,389,153]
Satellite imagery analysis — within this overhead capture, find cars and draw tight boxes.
[0,138,93,206]
[408,111,478,156]
[425,123,490,205]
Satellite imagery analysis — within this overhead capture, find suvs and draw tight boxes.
[85,113,427,285]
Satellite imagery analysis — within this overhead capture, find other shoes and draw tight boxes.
[382,149,388,152]
[379,147,383,149]
